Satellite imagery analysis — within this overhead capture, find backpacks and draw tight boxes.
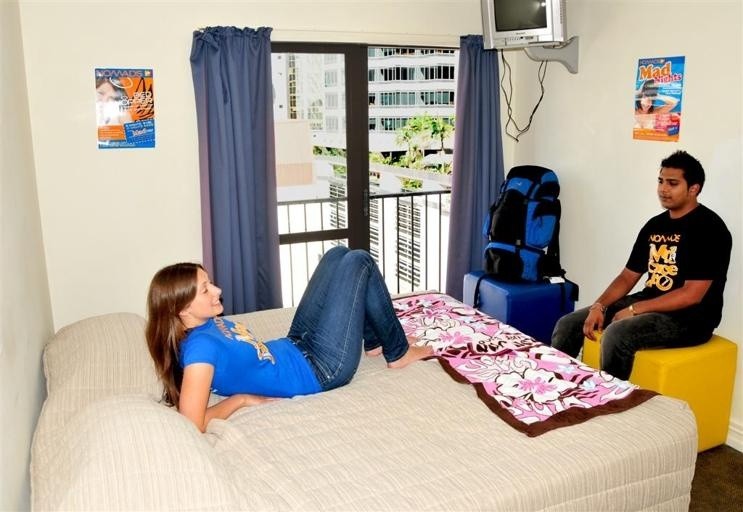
[483,164,564,283]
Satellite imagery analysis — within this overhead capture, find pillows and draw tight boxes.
[37,398,234,512]
[45,313,166,400]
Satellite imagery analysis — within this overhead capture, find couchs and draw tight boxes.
[463,270,579,348]
[582,330,738,453]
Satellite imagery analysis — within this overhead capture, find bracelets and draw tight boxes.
[590,302,608,313]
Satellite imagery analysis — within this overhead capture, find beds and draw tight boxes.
[28,289,700,512]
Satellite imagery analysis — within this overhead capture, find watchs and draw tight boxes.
[628,304,635,317]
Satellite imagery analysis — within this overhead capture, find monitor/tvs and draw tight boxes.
[481,0,568,50]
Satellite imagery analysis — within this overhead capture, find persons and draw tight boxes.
[145,245,436,434]
[95,78,127,122]
[550,151,733,380]
[634,81,680,128]
